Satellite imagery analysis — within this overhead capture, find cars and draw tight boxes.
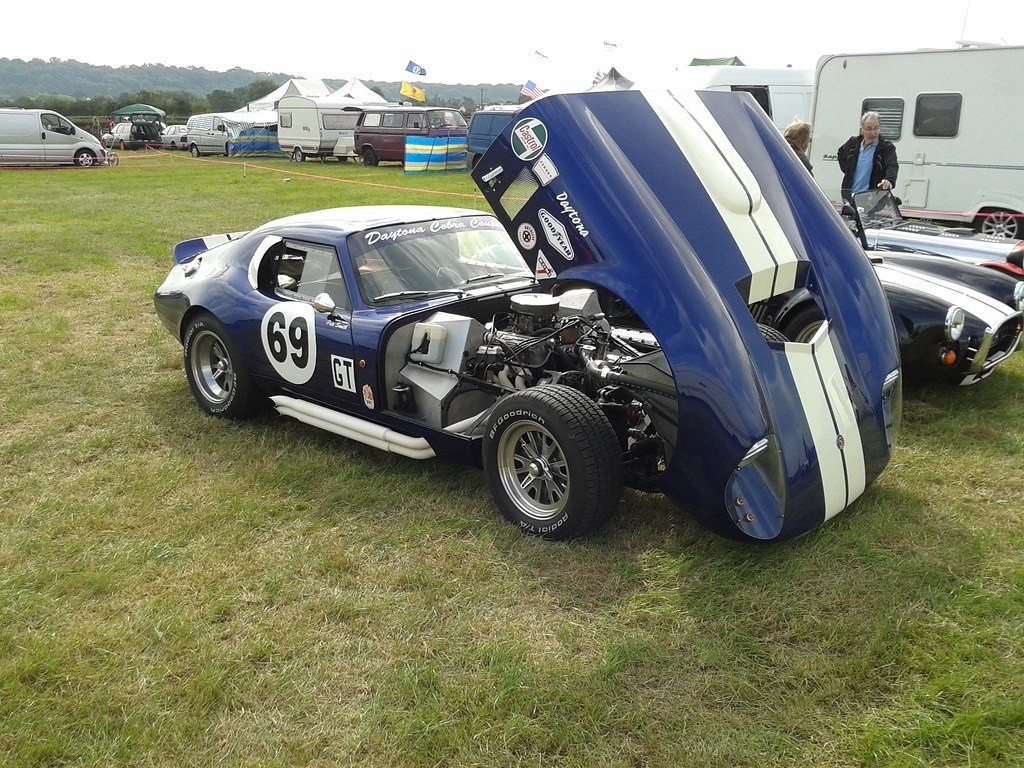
[101,123,160,151]
[160,125,188,150]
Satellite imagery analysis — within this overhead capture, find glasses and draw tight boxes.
[806,137,812,148]
[860,124,880,133]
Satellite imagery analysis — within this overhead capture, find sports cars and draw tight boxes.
[155,88,903,552]
[835,189,1024,397]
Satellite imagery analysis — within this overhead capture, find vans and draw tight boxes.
[0,107,107,168]
[353,106,470,167]
[187,115,233,158]
[467,104,523,170]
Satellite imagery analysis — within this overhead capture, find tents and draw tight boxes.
[234,76,387,113]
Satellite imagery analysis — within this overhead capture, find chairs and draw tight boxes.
[136,128,144,137]
[392,114,421,126]
[385,245,435,290]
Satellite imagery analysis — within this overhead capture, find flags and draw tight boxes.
[593,70,606,84]
[399,79,425,101]
[520,81,543,99]
[406,61,425,75]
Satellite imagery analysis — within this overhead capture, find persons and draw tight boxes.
[785,121,813,176]
[836,110,899,216]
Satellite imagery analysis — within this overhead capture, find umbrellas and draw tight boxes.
[112,103,166,117]
